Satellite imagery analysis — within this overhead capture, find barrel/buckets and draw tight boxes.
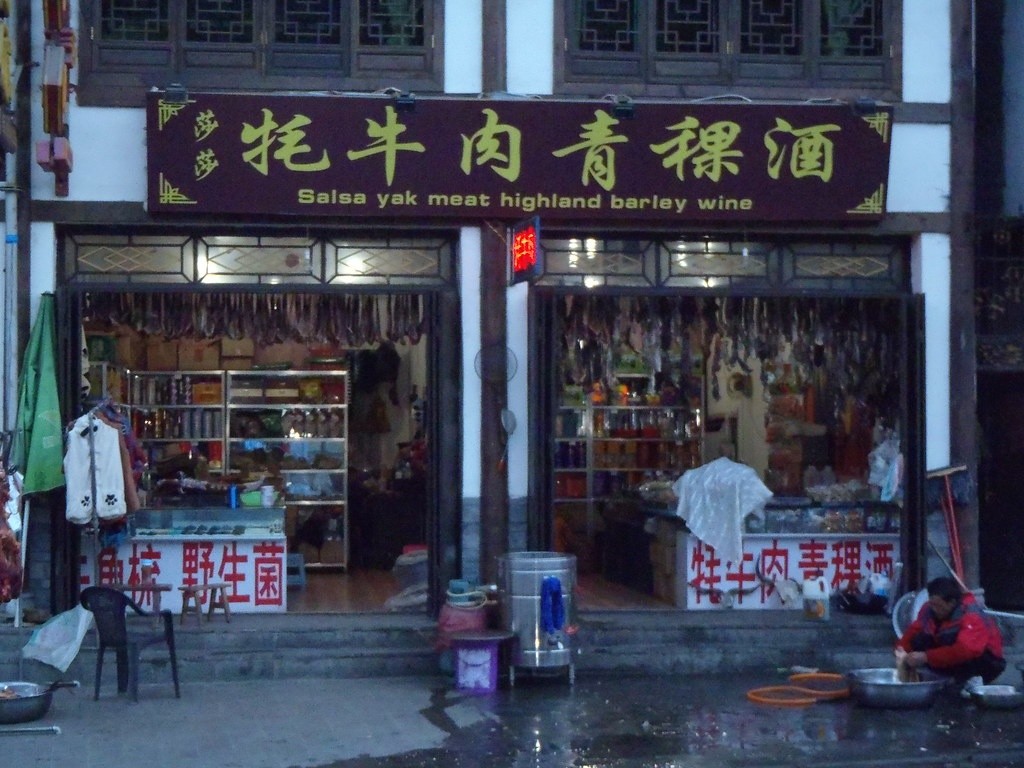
[454,640,498,694]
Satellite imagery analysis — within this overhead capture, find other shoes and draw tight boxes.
[962,677,984,697]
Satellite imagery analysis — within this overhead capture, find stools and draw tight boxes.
[287,554,307,588]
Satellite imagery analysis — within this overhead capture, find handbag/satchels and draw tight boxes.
[349,383,393,434]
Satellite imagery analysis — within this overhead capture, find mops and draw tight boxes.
[925,465,975,589]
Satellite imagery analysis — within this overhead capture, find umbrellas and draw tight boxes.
[5,290,65,628]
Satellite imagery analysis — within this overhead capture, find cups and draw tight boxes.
[260,485,275,507]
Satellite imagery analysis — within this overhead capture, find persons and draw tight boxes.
[895,575,1006,698]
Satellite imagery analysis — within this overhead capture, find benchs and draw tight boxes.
[178,583,232,627]
[103,583,173,628]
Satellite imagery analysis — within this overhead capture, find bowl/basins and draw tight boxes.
[847,668,947,710]
[892,591,917,641]
[967,684,1024,709]
[0,681,50,724]
[240,491,279,507]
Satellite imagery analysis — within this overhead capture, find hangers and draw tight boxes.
[84,399,108,418]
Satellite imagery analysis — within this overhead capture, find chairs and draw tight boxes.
[79,584,180,706]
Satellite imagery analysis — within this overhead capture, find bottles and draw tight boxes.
[802,572,830,623]
[228,483,237,509]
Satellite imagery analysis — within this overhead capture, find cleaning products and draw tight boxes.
[803,574,831,623]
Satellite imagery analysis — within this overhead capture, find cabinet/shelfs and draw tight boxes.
[82,362,130,422]
[591,371,706,552]
[549,394,590,551]
[226,369,351,575]
[132,369,226,477]
